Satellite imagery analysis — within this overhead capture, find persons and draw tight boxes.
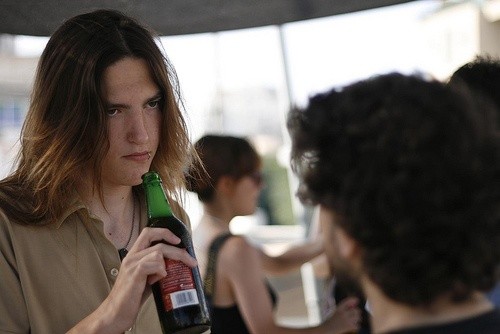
[0,8,213,334]
[185,134,360,334]
[284,53,500,334]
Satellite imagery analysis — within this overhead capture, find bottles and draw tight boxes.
[141,171,213,334]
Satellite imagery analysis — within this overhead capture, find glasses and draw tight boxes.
[247,173,261,185]
